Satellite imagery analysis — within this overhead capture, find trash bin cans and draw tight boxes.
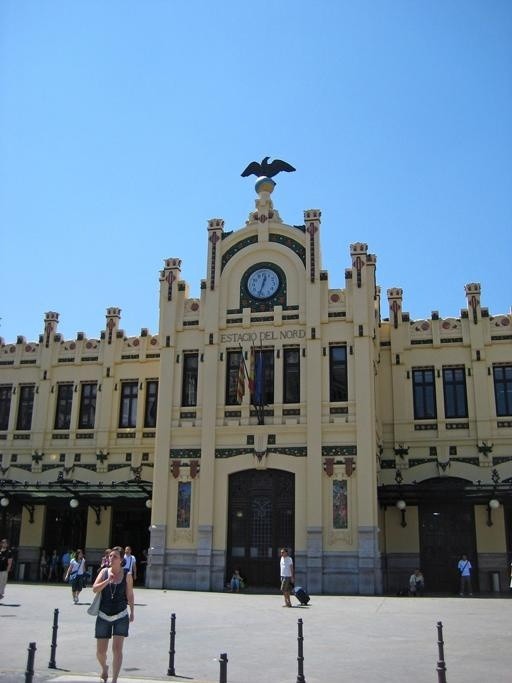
[18,562,30,580]
[491,572,500,592]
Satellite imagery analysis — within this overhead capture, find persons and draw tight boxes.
[409,568,426,596]
[0,537,13,600]
[40,547,137,604]
[229,569,244,593]
[456,554,474,599]
[92,546,135,683]
[278,548,295,608]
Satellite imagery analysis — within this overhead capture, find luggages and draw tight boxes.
[290,585,311,607]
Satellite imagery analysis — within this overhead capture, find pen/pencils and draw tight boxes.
[85,564,94,583]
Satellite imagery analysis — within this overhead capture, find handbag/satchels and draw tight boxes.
[86,591,103,616]
[68,570,79,582]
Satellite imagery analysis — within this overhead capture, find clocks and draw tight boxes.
[244,265,281,299]
